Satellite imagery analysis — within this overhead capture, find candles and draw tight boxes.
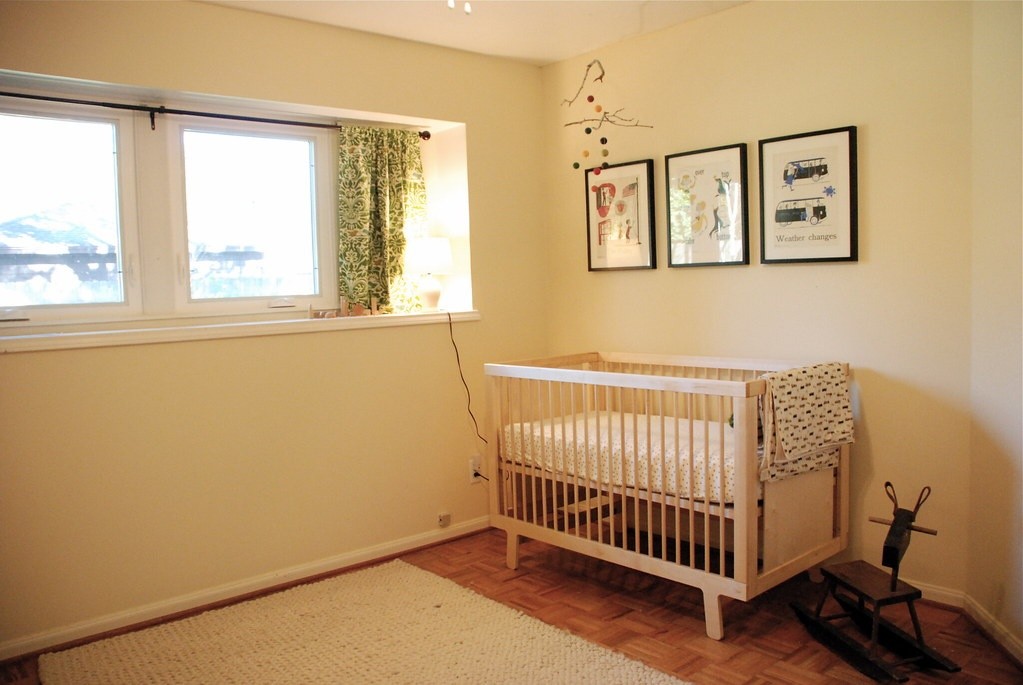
[420,288,441,310]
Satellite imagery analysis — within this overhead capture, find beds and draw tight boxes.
[482,353,852,641]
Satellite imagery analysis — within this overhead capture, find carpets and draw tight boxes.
[38,559,696,685]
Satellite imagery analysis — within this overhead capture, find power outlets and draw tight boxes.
[469,454,483,484]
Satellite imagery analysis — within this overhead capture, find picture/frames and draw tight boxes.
[585,158,657,272]
[664,142,749,266]
[758,126,858,263]
